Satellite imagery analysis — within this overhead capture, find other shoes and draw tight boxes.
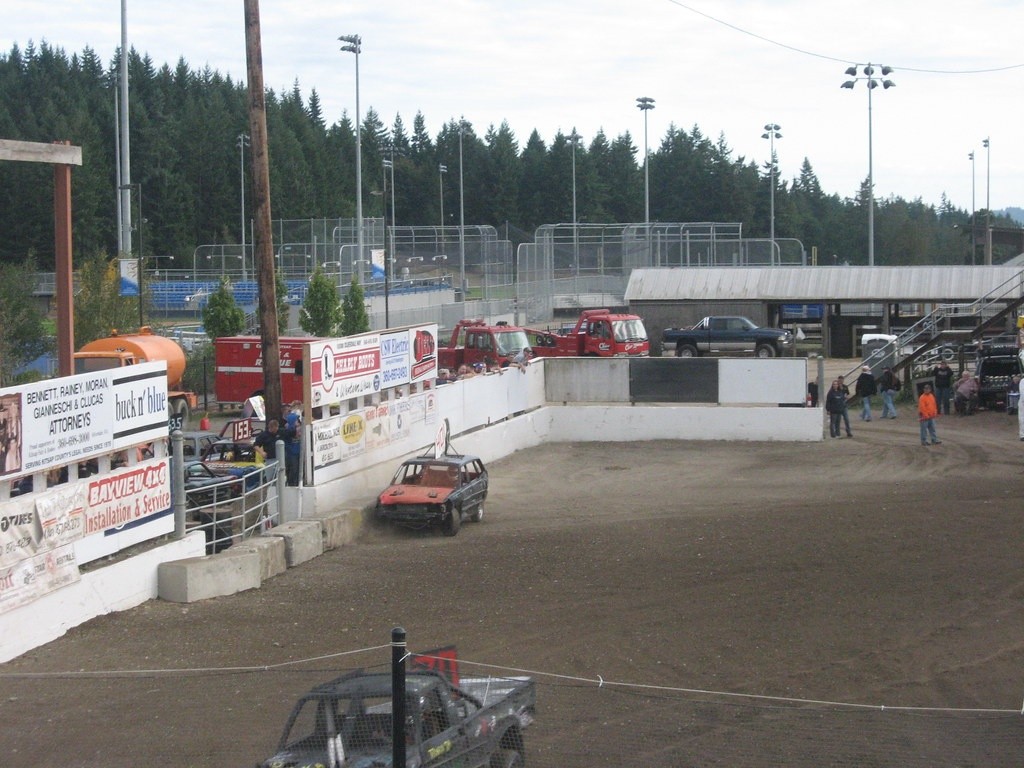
[932,439,942,445]
[923,442,931,445]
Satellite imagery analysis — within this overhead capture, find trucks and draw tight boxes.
[527,308,651,358]
[438,317,533,371]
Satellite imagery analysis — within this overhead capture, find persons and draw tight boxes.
[932,361,953,416]
[0,402,22,473]
[808,376,819,407]
[1008,376,1024,441]
[855,365,877,422]
[826,376,853,439]
[436,347,534,386]
[918,384,942,446]
[254,400,304,486]
[952,370,979,417]
[880,366,897,419]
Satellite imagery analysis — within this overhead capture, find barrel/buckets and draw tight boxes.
[201,508,233,556]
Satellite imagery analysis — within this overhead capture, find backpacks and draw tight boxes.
[889,372,901,390]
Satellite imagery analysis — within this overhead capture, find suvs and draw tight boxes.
[973,346,1024,410]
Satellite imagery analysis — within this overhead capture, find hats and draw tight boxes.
[881,366,889,370]
[860,366,872,374]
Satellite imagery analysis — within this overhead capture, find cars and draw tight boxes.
[10,431,277,520]
[373,454,491,533]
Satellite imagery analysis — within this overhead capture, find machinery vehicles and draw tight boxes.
[71,329,199,434]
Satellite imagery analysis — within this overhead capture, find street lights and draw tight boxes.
[636,96,654,266]
[377,143,406,298]
[761,123,783,265]
[145,255,174,275]
[235,133,252,283]
[967,150,974,266]
[438,164,450,264]
[840,63,896,264]
[459,128,473,303]
[340,35,363,297]
[120,182,142,329]
[565,128,582,276]
[982,138,990,267]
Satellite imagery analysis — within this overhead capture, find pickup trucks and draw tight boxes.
[258,671,540,768]
[661,315,794,358]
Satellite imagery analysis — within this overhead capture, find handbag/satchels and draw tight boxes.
[968,390,978,401]
